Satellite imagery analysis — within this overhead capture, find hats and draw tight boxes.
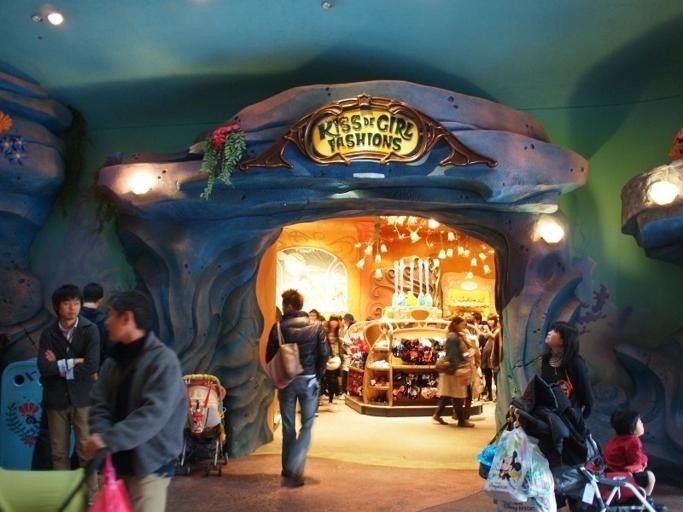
[343,314,356,323]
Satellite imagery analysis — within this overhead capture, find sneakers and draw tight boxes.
[318,396,322,405]
[339,393,346,400]
[433,414,448,425]
[458,419,475,427]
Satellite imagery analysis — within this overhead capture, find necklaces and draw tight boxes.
[554,357,562,363]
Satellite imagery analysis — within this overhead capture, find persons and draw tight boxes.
[37,283,111,507]
[298,310,354,416]
[541,321,594,418]
[599,409,656,505]
[433,311,498,426]
[265,290,330,486]
[86,289,189,512]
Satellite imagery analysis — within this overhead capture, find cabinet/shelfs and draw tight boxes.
[345,318,484,417]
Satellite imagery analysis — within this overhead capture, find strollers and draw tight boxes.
[176,373,229,478]
[507,397,669,512]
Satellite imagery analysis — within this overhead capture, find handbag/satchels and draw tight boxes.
[89,478,134,512]
[435,356,455,375]
[265,343,304,390]
[459,368,472,385]
